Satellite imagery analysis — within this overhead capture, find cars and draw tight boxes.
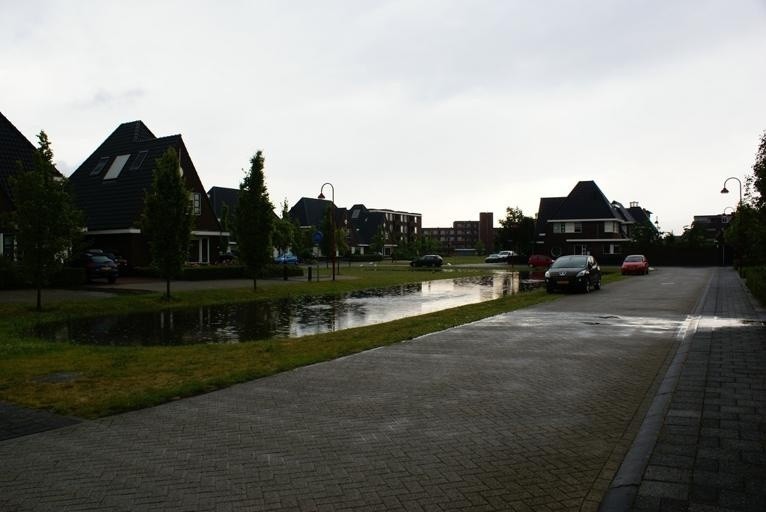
[528,255,601,293]
[86,255,118,283]
[485,251,519,263]
[276,255,298,264]
[410,255,443,267]
[621,255,649,275]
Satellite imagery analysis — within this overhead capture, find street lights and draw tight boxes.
[318,183,335,280]
[720,177,742,278]
